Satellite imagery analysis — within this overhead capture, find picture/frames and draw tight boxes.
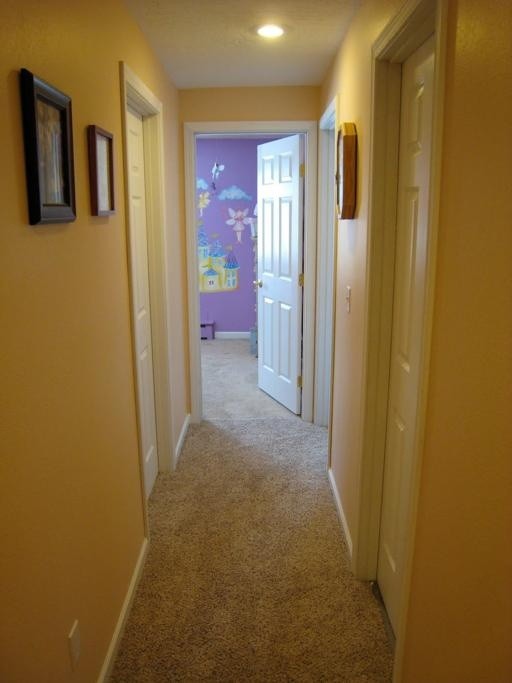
[17,68,77,226]
[87,123,116,218]
[334,123,358,219]
